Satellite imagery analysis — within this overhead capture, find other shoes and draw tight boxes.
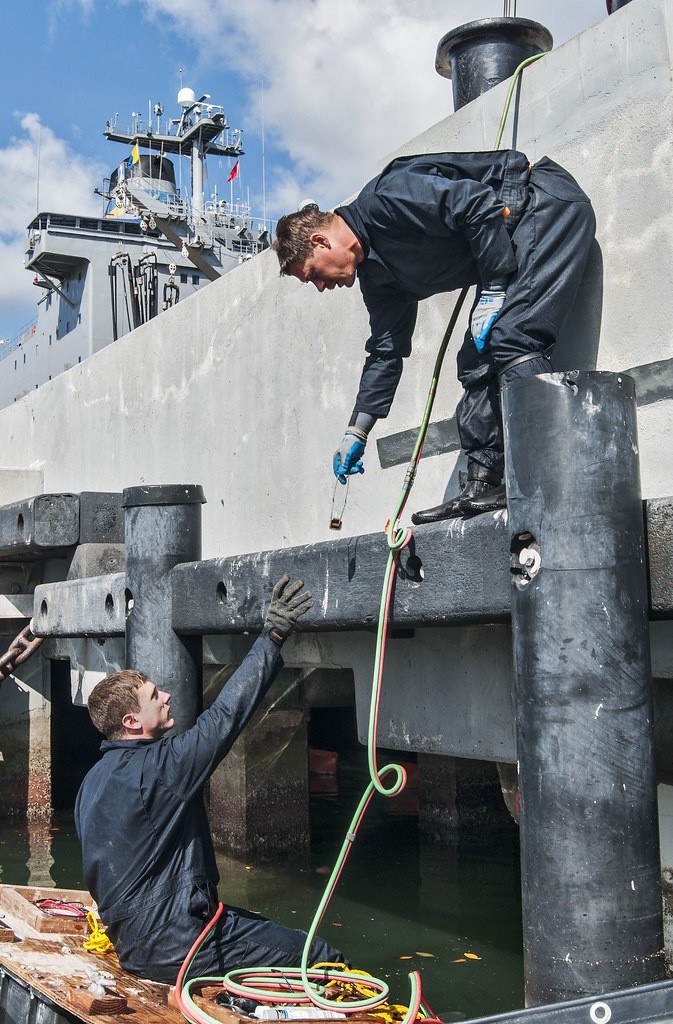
[460,482,507,513]
[411,463,500,525]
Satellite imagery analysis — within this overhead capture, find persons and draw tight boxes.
[72,573,349,991]
[274,147,597,526]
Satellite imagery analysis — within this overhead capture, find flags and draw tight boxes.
[227,160,238,182]
[127,143,139,169]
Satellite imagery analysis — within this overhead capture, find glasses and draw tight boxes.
[214,992,265,1018]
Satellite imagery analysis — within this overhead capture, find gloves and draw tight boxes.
[332,425,369,485]
[261,574,315,648]
[470,290,506,354]
